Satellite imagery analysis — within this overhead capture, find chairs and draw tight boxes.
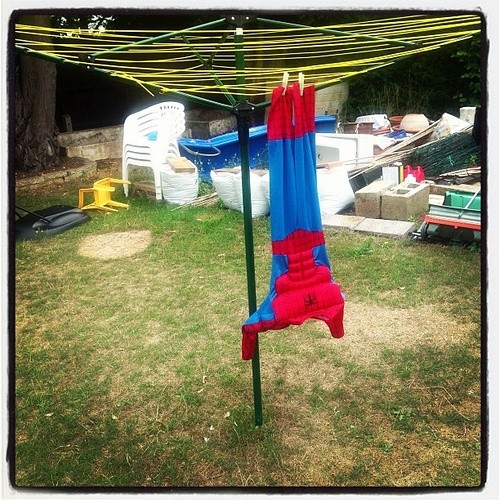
[77,177,131,213]
[121,100,187,202]
